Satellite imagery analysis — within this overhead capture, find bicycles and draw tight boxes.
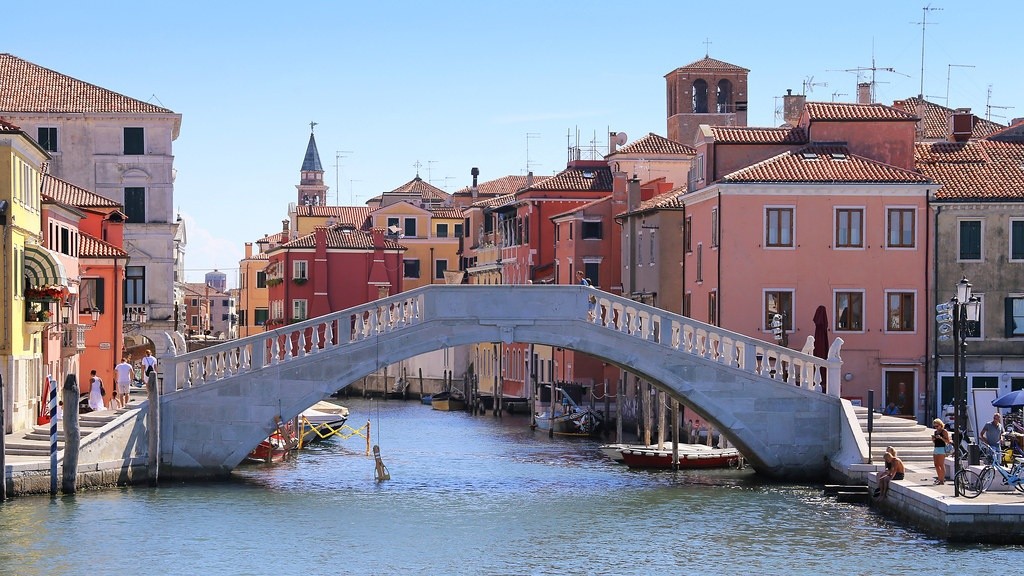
[975,449,1024,497]
[949,450,983,498]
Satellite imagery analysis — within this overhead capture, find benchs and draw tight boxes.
[969,466,1016,491]
[943,457,984,481]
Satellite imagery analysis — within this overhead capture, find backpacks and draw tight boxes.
[145,357,155,377]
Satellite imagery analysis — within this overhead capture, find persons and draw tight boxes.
[885,400,900,415]
[931,418,950,485]
[88,370,106,411]
[109,391,120,409]
[687,418,700,444]
[873,446,905,503]
[980,413,1005,465]
[141,350,159,397]
[576,271,596,322]
[114,358,134,409]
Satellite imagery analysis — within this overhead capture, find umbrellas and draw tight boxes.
[991,388,1024,408]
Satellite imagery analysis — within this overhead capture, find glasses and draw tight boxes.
[934,426,938,428]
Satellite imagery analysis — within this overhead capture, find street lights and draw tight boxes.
[947,274,981,498]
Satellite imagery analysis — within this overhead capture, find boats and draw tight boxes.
[421,395,433,405]
[432,391,465,411]
[249,419,297,463]
[598,439,741,469]
[298,420,322,448]
[534,397,599,437]
[298,401,350,442]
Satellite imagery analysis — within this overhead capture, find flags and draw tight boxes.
[37,374,54,426]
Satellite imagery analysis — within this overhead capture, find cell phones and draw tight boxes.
[931,435,936,438]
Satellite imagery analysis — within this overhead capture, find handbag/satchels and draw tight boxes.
[944,442,955,456]
[100,380,105,396]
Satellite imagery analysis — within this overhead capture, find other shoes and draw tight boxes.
[935,481,944,485]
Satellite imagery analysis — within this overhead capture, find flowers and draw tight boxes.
[25,282,70,301]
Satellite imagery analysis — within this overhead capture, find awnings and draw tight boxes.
[24,243,68,287]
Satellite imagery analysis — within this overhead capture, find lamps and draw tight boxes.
[44,299,71,332]
[78,306,100,328]
